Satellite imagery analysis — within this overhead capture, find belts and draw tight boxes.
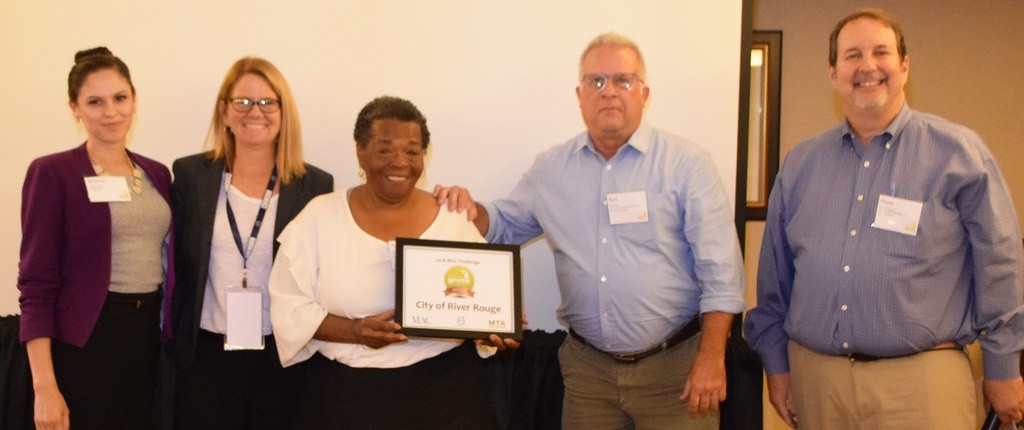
[838,342,966,363]
[567,317,701,367]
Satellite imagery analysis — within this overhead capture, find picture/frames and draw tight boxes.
[394,236,523,343]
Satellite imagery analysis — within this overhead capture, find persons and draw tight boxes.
[269,96,528,430]
[741,9,1024,430]
[431,34,747,430]
[17,47,174,430]
[168,57,336,430]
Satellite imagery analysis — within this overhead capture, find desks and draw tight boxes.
[0,316,763,430]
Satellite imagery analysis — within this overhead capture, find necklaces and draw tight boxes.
[92,155,143,196]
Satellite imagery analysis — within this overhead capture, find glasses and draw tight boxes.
[578,72,645,92]
[227,96,281,114]
